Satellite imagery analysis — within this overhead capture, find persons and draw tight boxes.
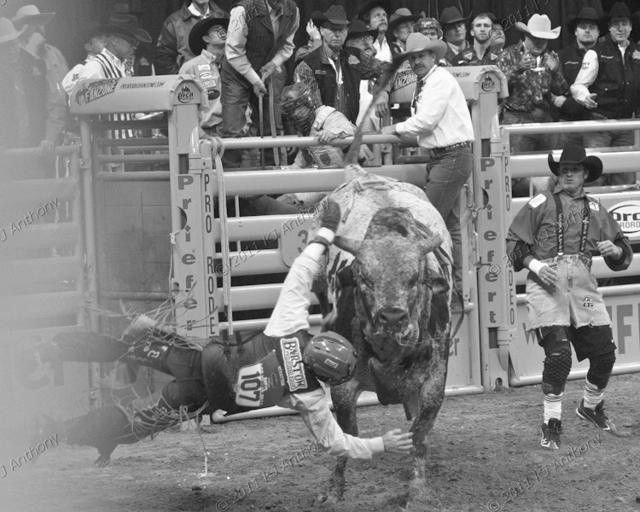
[0,3,640,313]
[31,197,415,461]
[505,144,633,450]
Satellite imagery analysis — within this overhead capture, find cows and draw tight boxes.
[305,51,459,510]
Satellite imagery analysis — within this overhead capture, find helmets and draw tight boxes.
[280,82,315,131]
[419,18,445,39]
[302,332,358,384]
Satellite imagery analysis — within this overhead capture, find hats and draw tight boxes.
[441,7,468,27]
[609,2,640,23]
[548,144,602,183]
[188,17,229,55]
[0,3,58,44]
[312,5,418,40]
[570,7,611,37]
[104,14,153,44]
[395,33,448,63]
[515,13,561,40]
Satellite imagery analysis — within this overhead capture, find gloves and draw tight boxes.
[307,198,340,254]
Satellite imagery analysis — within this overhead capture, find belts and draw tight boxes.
[428,142,468,157]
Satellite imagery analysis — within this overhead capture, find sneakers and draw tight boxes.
[576,397,612,431]
[540,418,563,450]
